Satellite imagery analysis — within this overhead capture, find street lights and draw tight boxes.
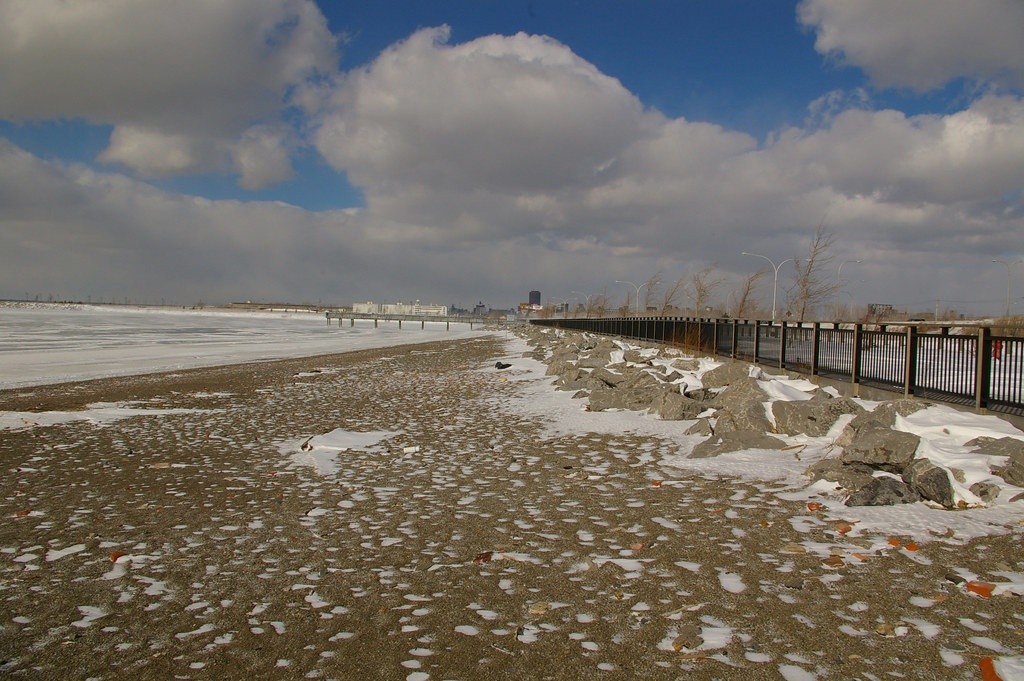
[740,252,813,324]
[570,290,606,315]
[615,280,662,318]
[992,259,1024,317]
[551,297,572,317]
[837,258,866,297]
